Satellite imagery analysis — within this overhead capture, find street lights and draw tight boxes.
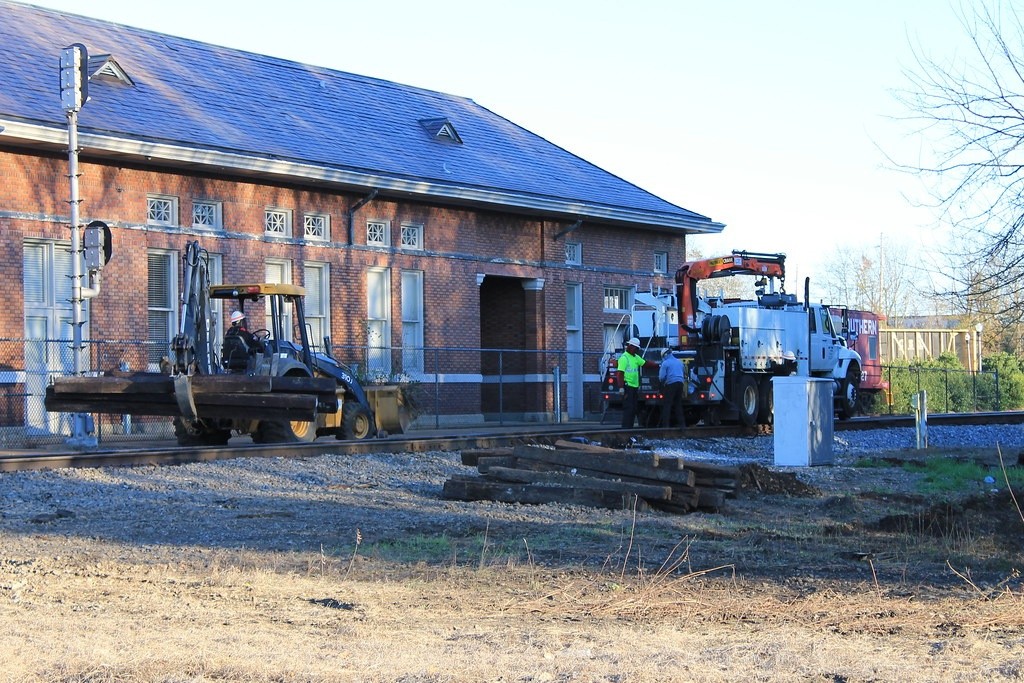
[975,322,983,372]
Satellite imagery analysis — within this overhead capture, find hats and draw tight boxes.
[626,338,641,348]
[231,311,245,323]
[660,348,669,356]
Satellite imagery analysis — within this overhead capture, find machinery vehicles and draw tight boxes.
[41,242,407,438]
[599,249,862,430]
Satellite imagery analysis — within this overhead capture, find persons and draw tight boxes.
[659,347,686,428]
[222,310,266,356]
[617,335,662,429]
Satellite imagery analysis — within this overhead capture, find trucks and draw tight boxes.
[830,303,883,412]
